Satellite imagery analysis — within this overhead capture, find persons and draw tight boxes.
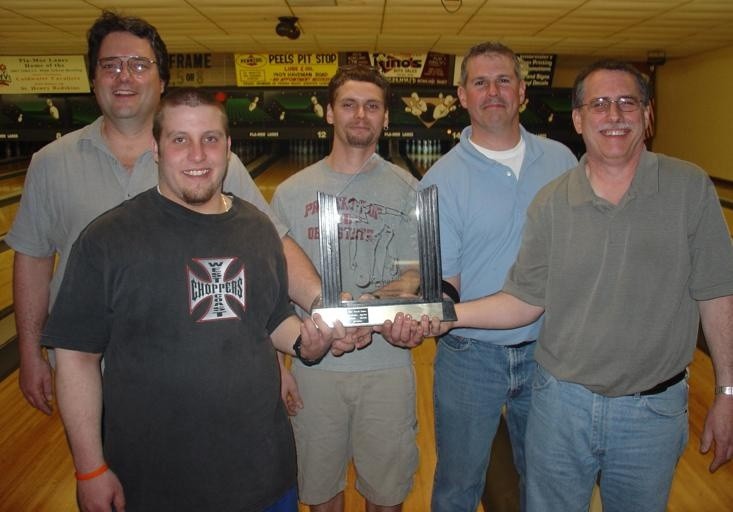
[38,85,346,509]
[0,7,354,416]
[421,58,733,511]
[270,63,420,510]
[419,39,580,511]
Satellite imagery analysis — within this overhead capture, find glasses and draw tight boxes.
[576,96,647,115]
[99,56,160,74]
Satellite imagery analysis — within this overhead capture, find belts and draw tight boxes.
[627,369,687,396]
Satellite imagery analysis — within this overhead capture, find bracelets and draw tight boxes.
[293,334,327,367]
[713,385,733,398]
[310,293,322,313]
[75,464,109,481]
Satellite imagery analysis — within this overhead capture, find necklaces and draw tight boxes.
[221,191,229,213]
